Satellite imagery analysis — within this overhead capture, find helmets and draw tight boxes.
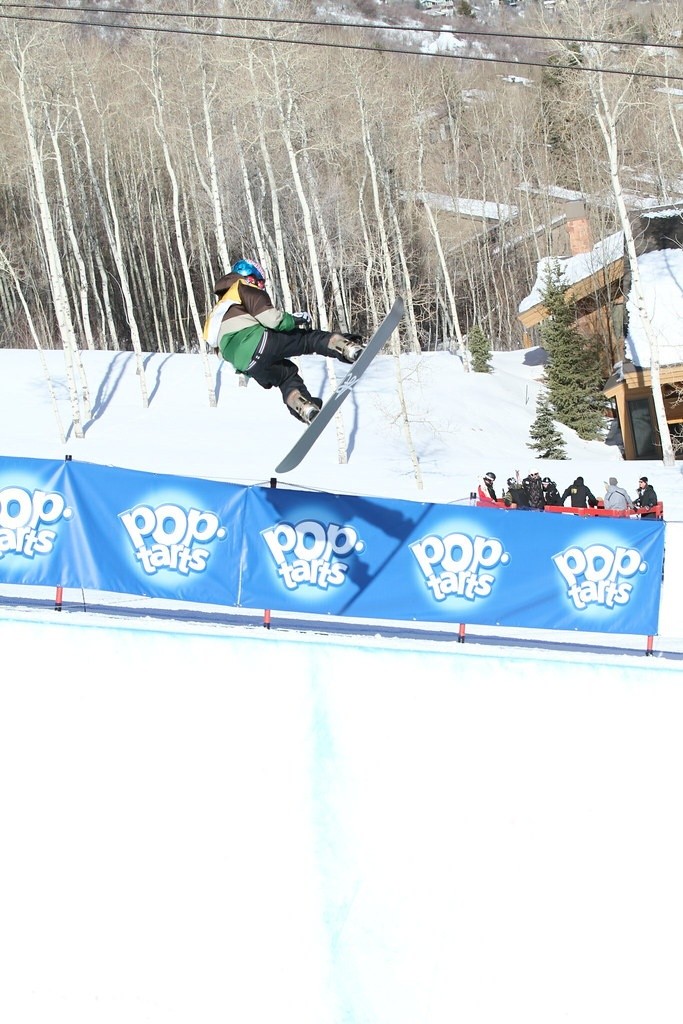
[485,472,495,480]
[232,260,266,282]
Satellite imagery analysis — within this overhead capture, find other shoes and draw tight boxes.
[287,389,321,422]
[328,334,363,362]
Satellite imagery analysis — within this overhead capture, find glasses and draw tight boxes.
[639,481,642,483]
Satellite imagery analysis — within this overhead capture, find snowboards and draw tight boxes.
[275,295,406,474]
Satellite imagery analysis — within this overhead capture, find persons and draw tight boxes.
[561,477,598,515]
[604,478,638,517]
[204,258,366,424]
[632,477,657,518]
[478,472,497,502]
[503,473,564,512]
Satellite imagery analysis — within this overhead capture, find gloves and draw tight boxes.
[633,506,638,512]
[291,312,312,330]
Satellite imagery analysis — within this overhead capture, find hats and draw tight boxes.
[609,478,618,485]
[640,477,648,483]
[542,478,551,484]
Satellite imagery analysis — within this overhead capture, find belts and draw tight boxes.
[246,332,268,371]
